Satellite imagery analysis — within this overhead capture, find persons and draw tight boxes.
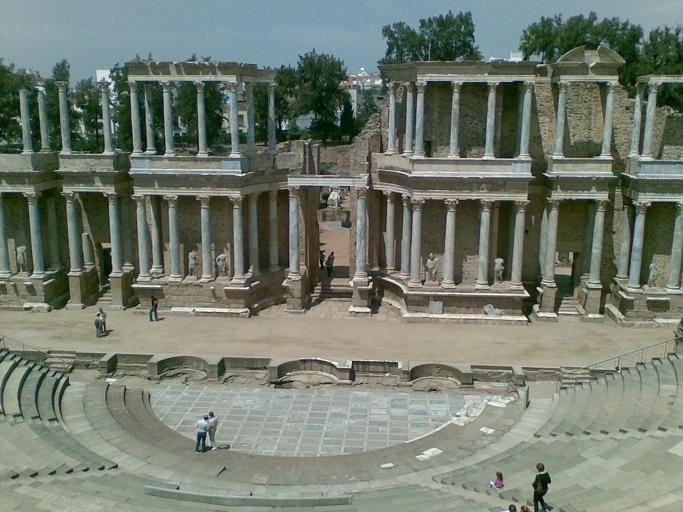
[319,250,325,271]
[186,249,198,277]
[206,409,219,449]
[647,261,658,288]
[93,312,103,339]
[488,471,504,490]
[93,306,108,336]
[214,251,228,278]
[148,294,158,322]
[424,251,439,283]
[493,257,504,283]
[328,251,335,273]
[14,244,29,273]
[324,256,333,277]
[193,414,211,454]
[531,461,552,511]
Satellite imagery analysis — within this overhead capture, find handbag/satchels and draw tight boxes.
[535,474,544,494]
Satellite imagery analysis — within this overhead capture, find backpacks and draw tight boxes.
[94,319,99,326]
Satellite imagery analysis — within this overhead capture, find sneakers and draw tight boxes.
[207,444,217,450]
